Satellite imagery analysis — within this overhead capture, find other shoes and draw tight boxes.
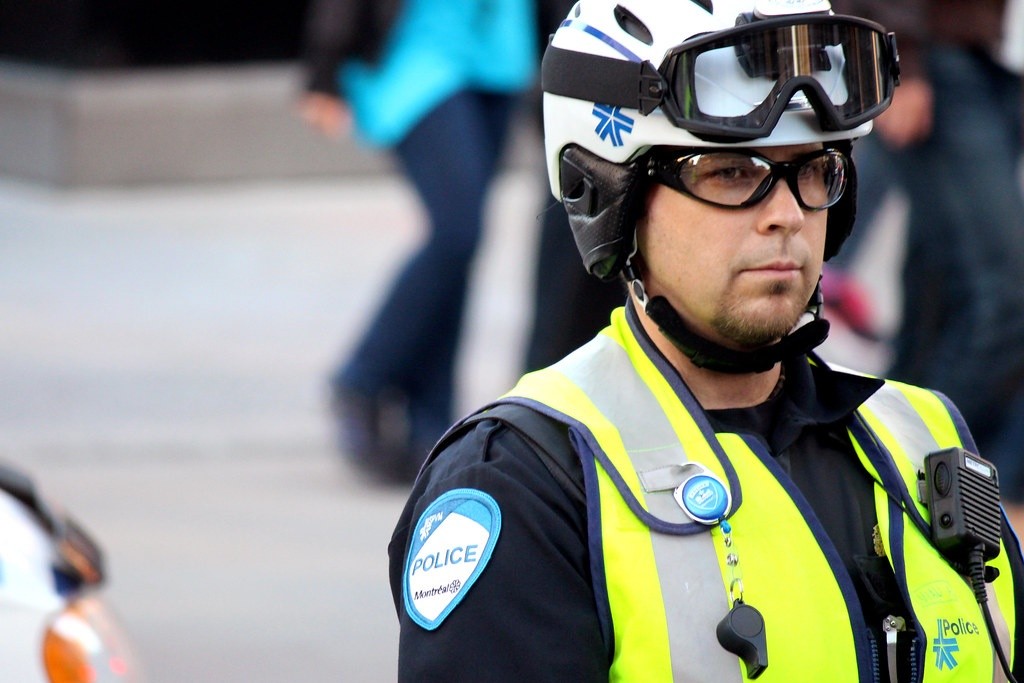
[403,437,454,483]
[337,375,413,485]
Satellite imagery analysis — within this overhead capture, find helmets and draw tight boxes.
[543,0,901,282]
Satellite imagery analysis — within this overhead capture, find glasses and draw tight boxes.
[645,147,849,212]
[542,13,901,143]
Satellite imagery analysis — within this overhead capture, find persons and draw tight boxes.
[1,468,125,683]
[385,0,1023,683]
[303,0,569,485]
